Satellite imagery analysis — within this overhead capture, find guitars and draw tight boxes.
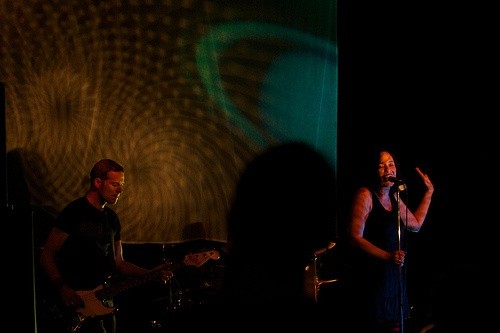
[55,248,221,333]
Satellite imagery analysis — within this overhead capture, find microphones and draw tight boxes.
[385,175,404,185]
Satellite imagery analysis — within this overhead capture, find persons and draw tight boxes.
[174,139,392,332]
[42,159,166,333]
[348,149,435,333]
